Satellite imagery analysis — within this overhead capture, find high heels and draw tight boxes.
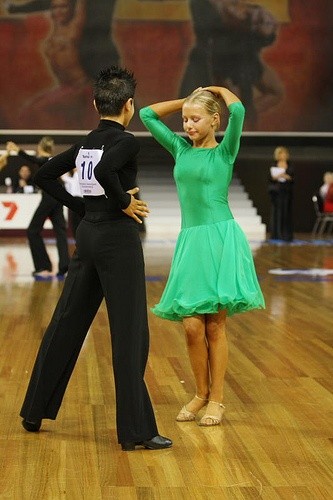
[176,394,209,422]
[199,400,226,426]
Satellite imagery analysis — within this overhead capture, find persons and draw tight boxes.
[263,147,295,244]
[0,137,70,277]
[316,171,333,215]
[139,86,267,425]
[20,66,174,451]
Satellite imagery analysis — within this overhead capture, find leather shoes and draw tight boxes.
[121,434,173,451]
[22,417,42,433]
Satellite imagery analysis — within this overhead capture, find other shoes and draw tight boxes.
[56,265,69,277]
[34,264,52,273]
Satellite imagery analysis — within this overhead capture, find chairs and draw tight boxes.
[311,195,333,237]
